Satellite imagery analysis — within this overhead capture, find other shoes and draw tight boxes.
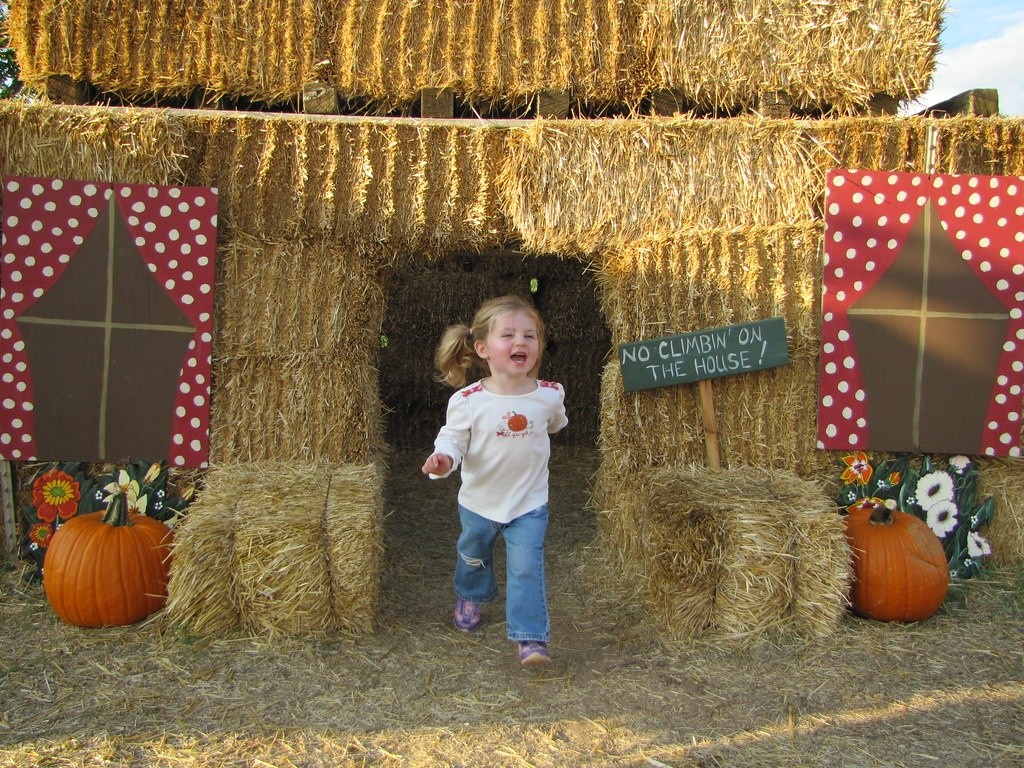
[453,597,481,631]
[516,641,552,669]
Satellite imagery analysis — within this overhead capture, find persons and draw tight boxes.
[419,295,570,666]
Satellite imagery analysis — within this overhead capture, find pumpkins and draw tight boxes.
[43,485,178,627]
[842,506,949,622]
